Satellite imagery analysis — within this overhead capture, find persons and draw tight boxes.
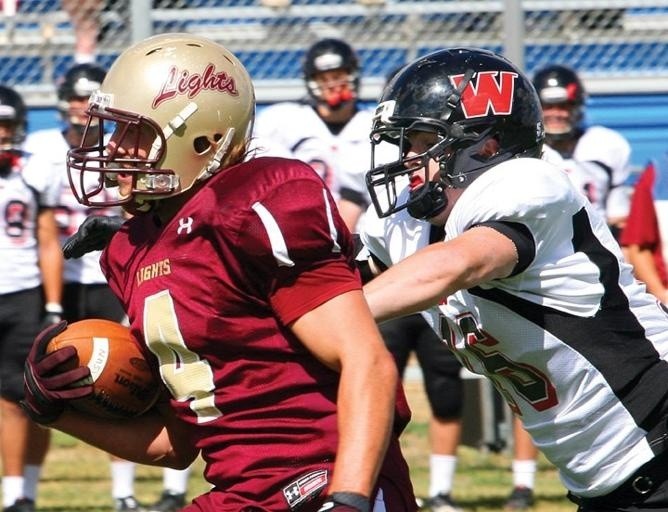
[1,82,68,511]
[621,150,667,307]
[359,46,667,512]
[13,35,423,512]
[334,65,464,512]
[496,60,636,511]
[20,63,152,512]
[245,37,419,233]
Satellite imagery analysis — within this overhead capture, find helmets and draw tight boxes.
[66,32,255,214]
[534,64,586,143]
[365,47,546,220]
[304,39,362,111]
[56,63,107,135]
[0,85,28,157]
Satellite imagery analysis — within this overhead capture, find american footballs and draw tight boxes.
[46,319,157,419]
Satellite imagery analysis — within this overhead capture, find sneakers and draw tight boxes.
[502,486,534,511]
[419,492,457,512]
[115,496,153,512]
[151,489,187,512]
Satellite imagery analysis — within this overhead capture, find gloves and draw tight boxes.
[61,217,129,260]
[37,303,65,335]
[20,319,95,426]
